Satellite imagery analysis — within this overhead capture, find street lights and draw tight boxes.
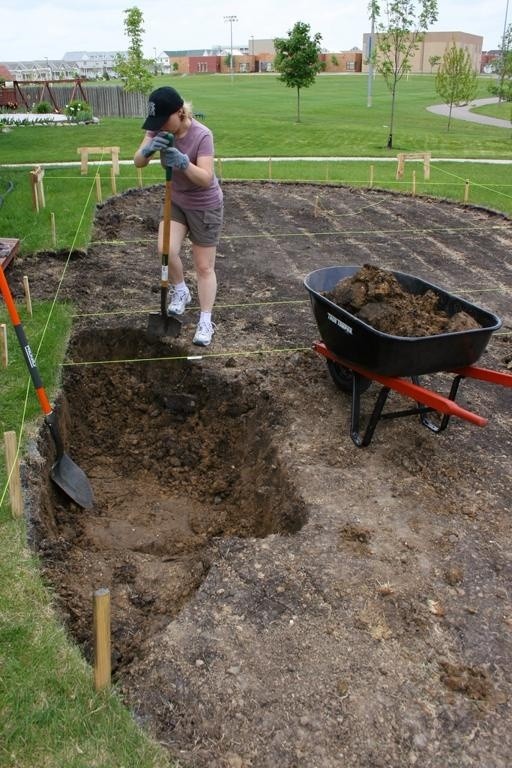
[44,56,48,66]
[152,45,158,58]
[222,14,241,82]
[249,33,255,71]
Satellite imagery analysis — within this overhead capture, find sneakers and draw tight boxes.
[192,320,217,346]
[167,287,191,315]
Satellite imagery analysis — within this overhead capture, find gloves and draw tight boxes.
[160,147,189,169]
[142,131,170,157]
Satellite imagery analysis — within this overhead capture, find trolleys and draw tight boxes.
[301,262,512,446]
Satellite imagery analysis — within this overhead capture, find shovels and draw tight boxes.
[0,265,95,509]
[145,132,183,347]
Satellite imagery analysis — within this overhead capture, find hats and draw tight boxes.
[140,86,183,130]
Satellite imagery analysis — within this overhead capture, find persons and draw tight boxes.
[133,85,224,347]
